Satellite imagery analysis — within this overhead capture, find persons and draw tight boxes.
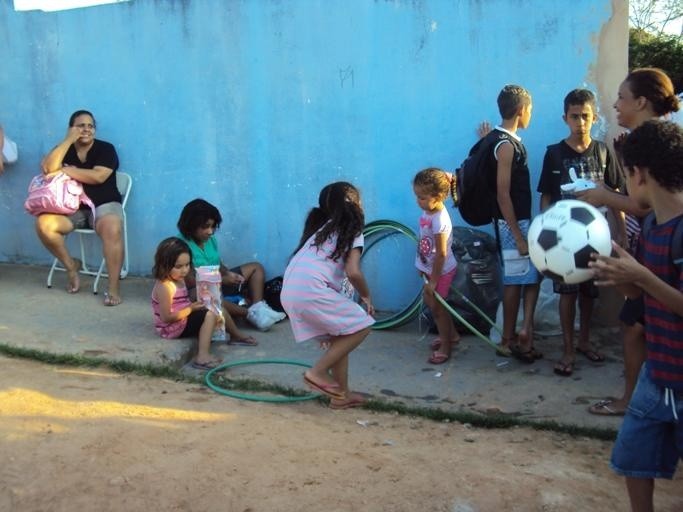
[0,125,5,176]
[151,236,257,373]
[35,111,124,307]
[536,89,630,377]
[571,68,680,418]
[175,199,286,332]
[413,167,459,365]
[279,182,377,410]
[588,120,683,512]
[477,84,545,363]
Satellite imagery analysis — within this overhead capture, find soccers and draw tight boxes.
[528,199,613,285]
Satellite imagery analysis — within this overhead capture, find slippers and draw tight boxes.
[228,335,258,347]
[192,357,226,372]
[588,400,626,416]
[329,392,364,410]
[427,335,460,364]
[303,371,346,400]
[495,341,606,376]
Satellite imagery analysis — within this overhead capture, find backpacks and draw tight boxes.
[456,128,525,227]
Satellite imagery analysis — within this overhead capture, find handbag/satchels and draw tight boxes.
[24,166,97,229]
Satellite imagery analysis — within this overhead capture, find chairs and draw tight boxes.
[47,171,133,295]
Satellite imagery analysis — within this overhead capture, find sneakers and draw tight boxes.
[246,301,286,333]
